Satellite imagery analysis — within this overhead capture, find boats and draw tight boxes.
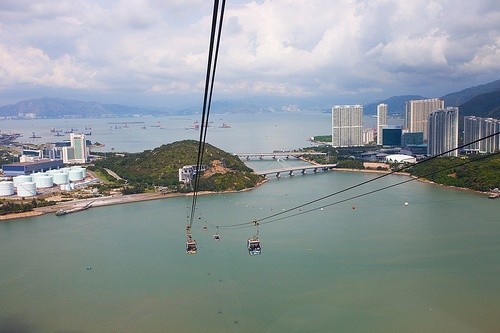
[53,202,92,217]
[25,118,231,149]
[488,192,500,199]
